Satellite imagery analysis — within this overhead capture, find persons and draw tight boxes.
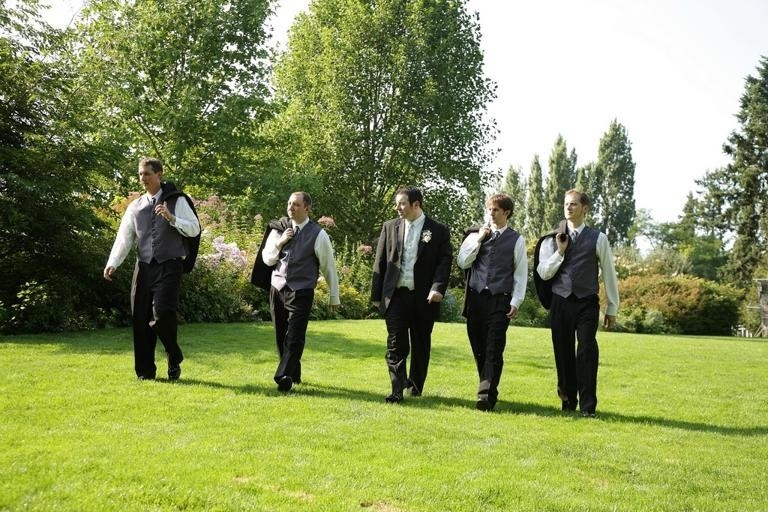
[369,184,454,403]
[261,190,342,392]
[536,188,621,419]
[456,194,529,411]
[102,157,203,382]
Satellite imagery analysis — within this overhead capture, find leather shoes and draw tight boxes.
[582,411,595,417]
[292,377,300,384]
[386,391,403,401]
[477,398,492,411]
[278,376,292,391]
[168,363,181,381]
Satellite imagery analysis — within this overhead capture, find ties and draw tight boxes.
[573,231,578,242]
[493,231,500,239]
[294,227,299,236]
[404,224,414,249]
[152,198,155,207]
